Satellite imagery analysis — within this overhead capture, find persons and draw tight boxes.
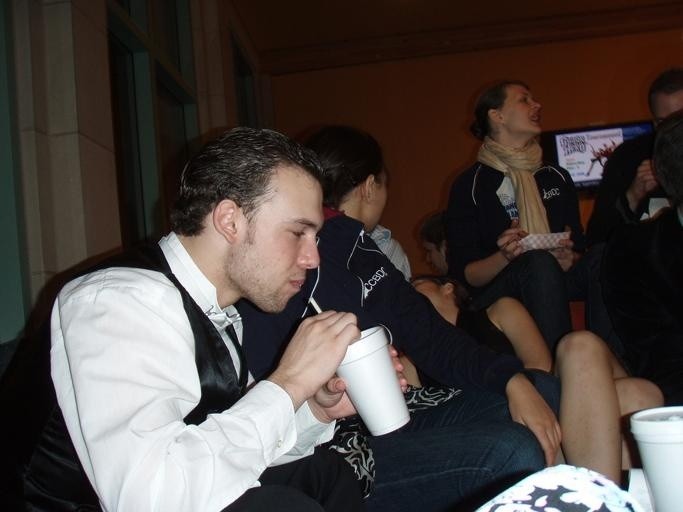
[408,80,664,487]
[236,122,560,512]
[586,66,682,405]
[0,128,408,512]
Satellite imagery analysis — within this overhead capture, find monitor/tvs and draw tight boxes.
[539,119,654,188]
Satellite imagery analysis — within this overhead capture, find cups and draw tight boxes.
[330,327,410,436]
[631,405,683,512]
